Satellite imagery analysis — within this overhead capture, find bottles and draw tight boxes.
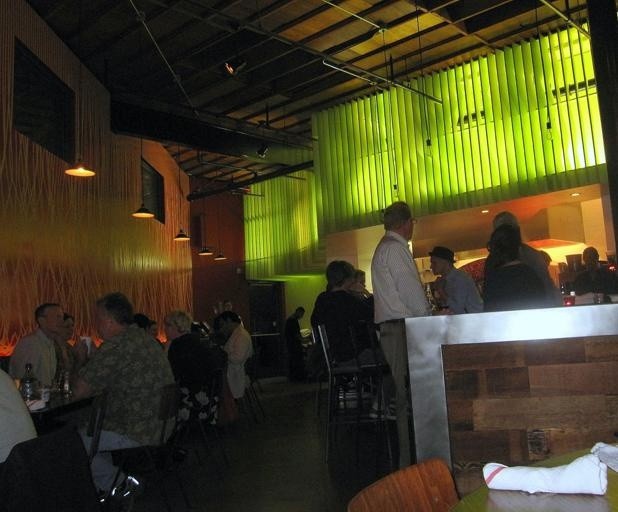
[21,363,39,400]
[72,334,88,365]
[40,374,73,402]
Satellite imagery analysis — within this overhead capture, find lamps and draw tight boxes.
[193,118,214,261]
[367,78,390,221]
[66,1,96,180]
[255,139,269,161]
[411,0,436,161]
[129,26,154,223]
[223,50,246,74]
[378,25,404,200]
[534,1,556,141]
[213,148,226,263]
[171,71,189,245]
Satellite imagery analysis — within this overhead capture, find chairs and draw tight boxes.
[1,306,290,512]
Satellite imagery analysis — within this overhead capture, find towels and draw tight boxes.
[474,442,614,498]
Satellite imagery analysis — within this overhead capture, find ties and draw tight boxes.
[51,337,65,382]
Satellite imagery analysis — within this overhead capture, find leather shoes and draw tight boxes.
[94,475,140,506]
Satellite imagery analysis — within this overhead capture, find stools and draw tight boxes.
[315,324,412,501]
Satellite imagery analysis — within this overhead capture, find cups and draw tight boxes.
[594,288,606,304]
[563,294,576,305]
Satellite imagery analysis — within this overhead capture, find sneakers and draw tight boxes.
[368,407,384,419]
[386,404,397,421]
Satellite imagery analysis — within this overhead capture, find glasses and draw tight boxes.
[411,218,416,224]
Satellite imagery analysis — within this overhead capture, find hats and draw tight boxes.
[428,246,455,264]
